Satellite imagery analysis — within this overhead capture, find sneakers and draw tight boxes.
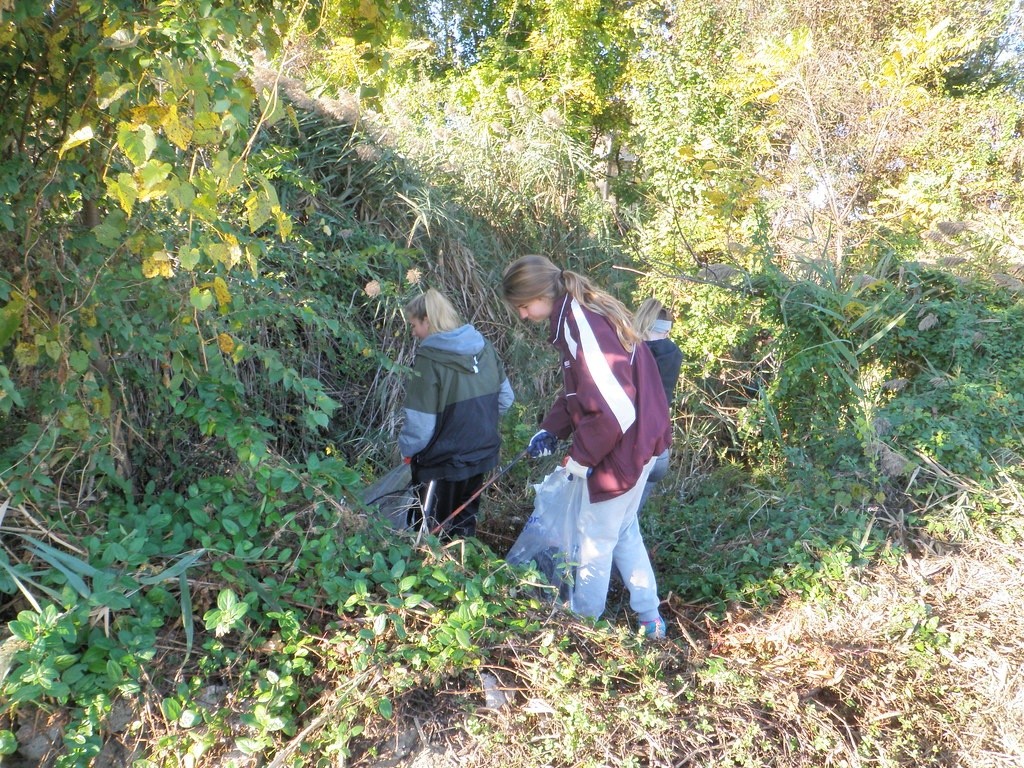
[631,612,666,640]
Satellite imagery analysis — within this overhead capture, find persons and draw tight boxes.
[503,254,671,642]
[397,289,514,539]
[631,296,683,515]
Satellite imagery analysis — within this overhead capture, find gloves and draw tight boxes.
[562,456,592,481]
[527,428,559,458]
